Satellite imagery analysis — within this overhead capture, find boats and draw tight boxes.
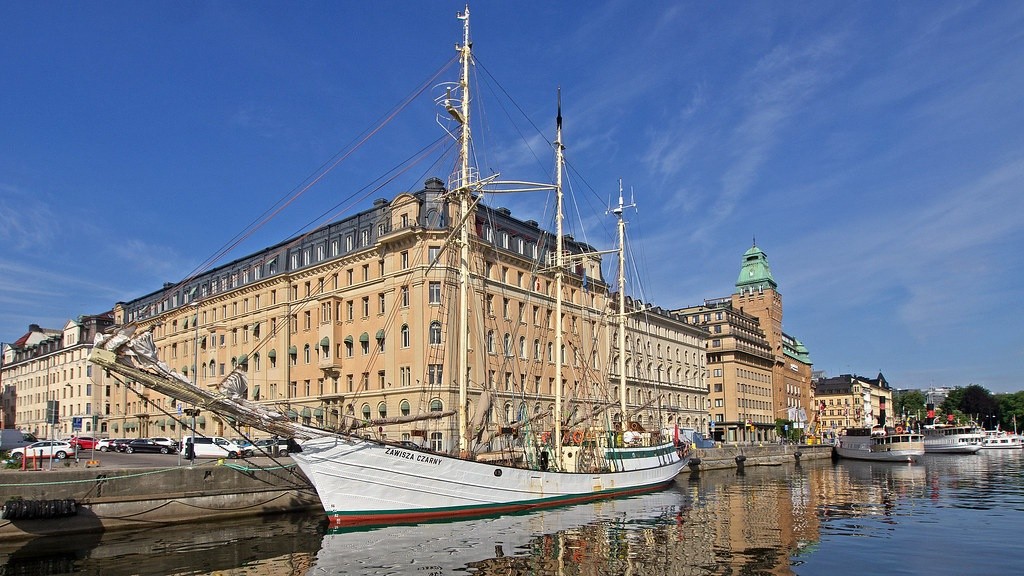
[981,423,1024,449]
[921,421,989,453]
[834,423,926,464]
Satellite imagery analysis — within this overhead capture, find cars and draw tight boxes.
[117,437,178,455]
[5,439,79,462]
[66,436,100,450]
[96,438,131,454]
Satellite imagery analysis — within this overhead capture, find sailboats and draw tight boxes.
[85,2,698,535]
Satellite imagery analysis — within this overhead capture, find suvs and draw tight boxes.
[148,436,178,451]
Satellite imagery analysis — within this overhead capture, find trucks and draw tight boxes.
[0,428,43,454]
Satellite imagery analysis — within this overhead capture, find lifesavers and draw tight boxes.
[541,432,550,443]
[573,431,583,443]
[631,423,637,430]
[563,434,570,444]
[614,424,620,429]
[1008,432,1014,434]
[896,427,902,434]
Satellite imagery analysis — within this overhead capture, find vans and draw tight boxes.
[181,436,242,460]
[243,438,290,458]
